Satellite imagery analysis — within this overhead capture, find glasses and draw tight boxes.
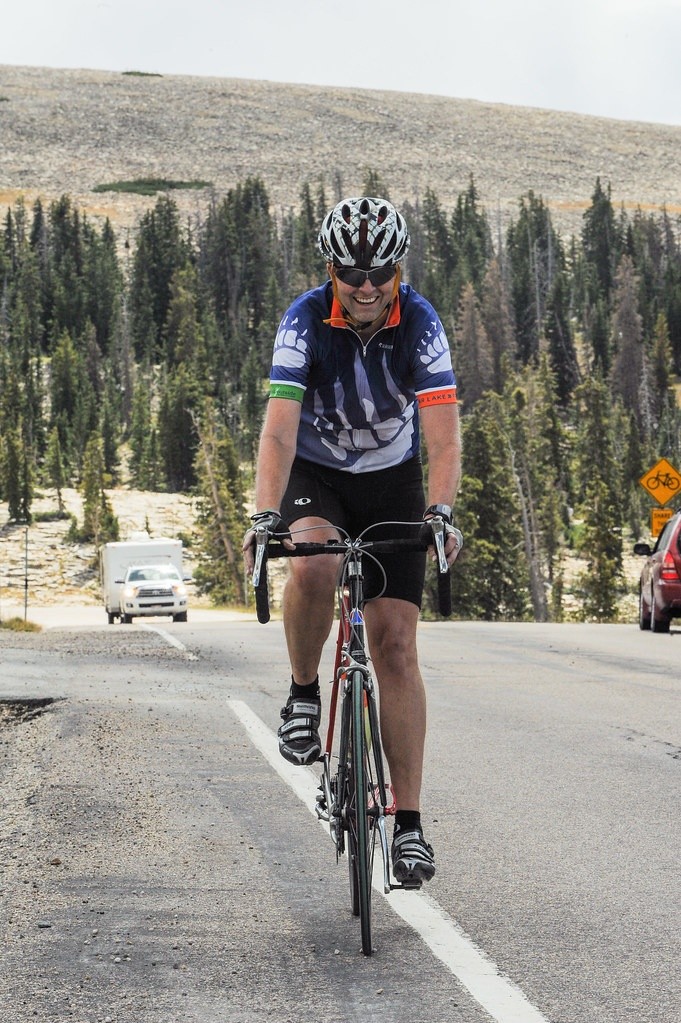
[332,265,396,287]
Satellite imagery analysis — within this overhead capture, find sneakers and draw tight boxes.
[277,694,322,765]
[390,828,435,890]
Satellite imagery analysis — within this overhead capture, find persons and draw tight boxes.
[243,197,463,882]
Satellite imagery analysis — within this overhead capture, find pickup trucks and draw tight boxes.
[98,537,193,625]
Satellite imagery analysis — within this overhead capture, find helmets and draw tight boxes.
[318,197,411,266]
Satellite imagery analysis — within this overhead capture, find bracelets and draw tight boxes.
[423,504,454,525]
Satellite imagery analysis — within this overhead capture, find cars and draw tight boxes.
[633,507,681,633]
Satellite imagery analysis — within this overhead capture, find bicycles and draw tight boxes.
[250,515,453,957]
